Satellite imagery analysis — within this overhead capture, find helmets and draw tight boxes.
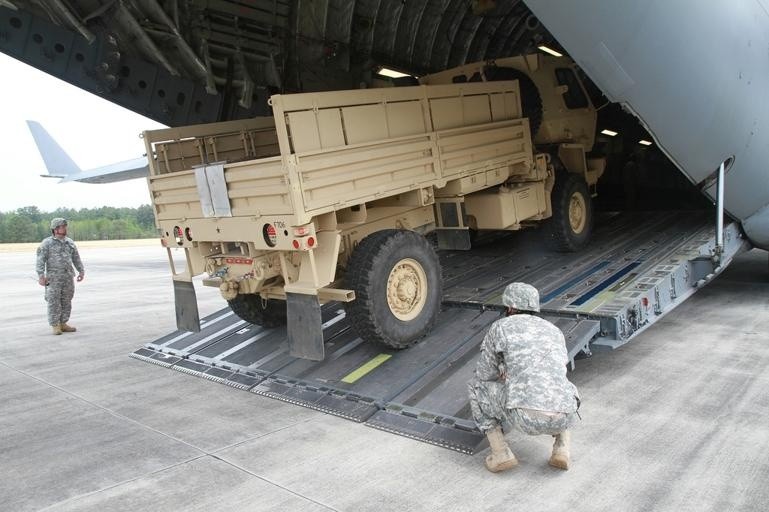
[50,218,68,230]
[501,282,540,314]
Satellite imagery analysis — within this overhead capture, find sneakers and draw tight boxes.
[60,323,77,332]
[52,324,63,335]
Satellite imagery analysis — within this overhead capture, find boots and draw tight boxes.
[485,424,518,473]
[548,429,571,471]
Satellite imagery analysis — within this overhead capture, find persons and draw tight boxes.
[467,282,580,473]
[36,218,84,336]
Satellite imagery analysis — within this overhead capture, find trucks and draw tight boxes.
[135,48,611,354]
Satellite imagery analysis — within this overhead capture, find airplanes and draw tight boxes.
[0,2,767,459]
